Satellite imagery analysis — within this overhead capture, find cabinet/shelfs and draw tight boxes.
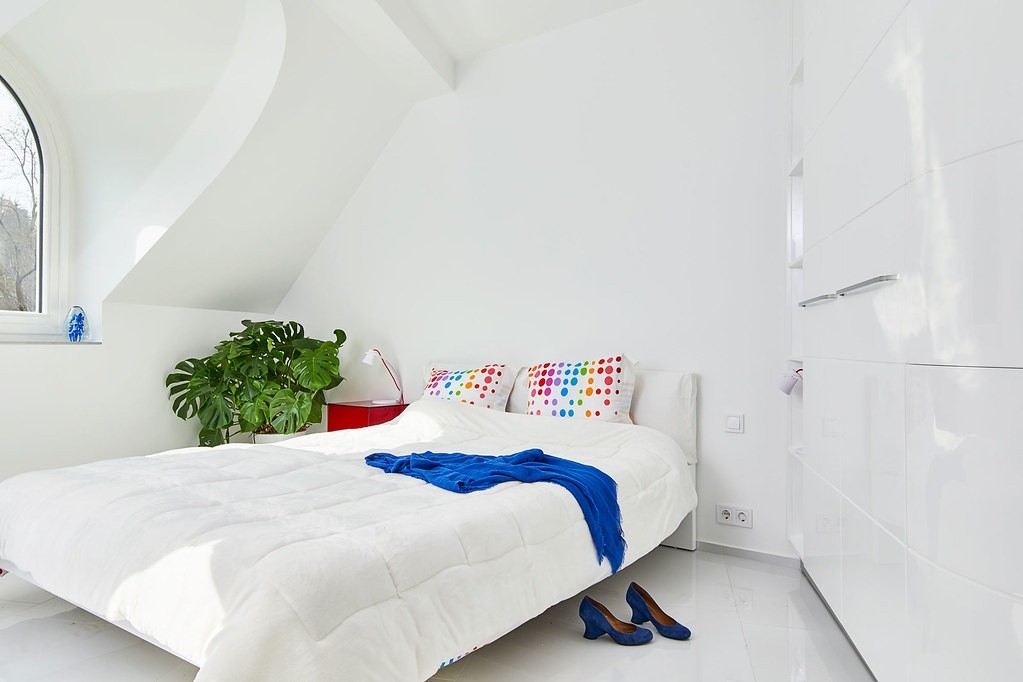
[781,0,1023,682]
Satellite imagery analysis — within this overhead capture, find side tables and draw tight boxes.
[326,400,410,433]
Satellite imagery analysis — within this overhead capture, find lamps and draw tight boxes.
[776,369,803,395]
[362,348,404,405]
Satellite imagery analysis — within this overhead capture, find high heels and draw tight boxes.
[626,582,691,640]
[579,596,653,645]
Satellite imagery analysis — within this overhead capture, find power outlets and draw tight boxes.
[716,504,753,528]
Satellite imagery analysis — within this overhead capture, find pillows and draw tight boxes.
[421,363,523,413]
[527,354,635,424]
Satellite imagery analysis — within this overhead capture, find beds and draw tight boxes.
[0,367,699,682]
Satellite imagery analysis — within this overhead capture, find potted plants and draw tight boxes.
[165,319,347,447]
